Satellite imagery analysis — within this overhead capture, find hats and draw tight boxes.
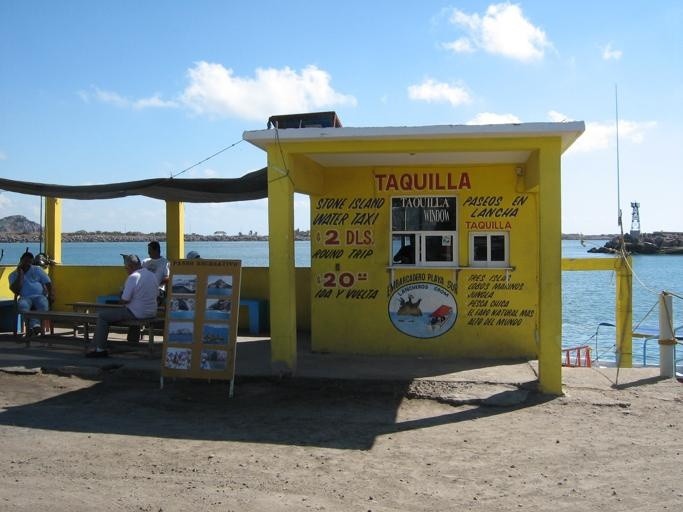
[187,251,199,259]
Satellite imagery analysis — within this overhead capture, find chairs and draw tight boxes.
[14,274,54,335]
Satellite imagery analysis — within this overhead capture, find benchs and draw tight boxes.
[23,295,261,352]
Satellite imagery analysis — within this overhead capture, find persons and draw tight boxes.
[85,254,160,360]
[127,241,170,346]
[8,252,55,339]
[393,235,415,263]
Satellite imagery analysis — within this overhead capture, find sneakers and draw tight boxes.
[85,348,109,359]
